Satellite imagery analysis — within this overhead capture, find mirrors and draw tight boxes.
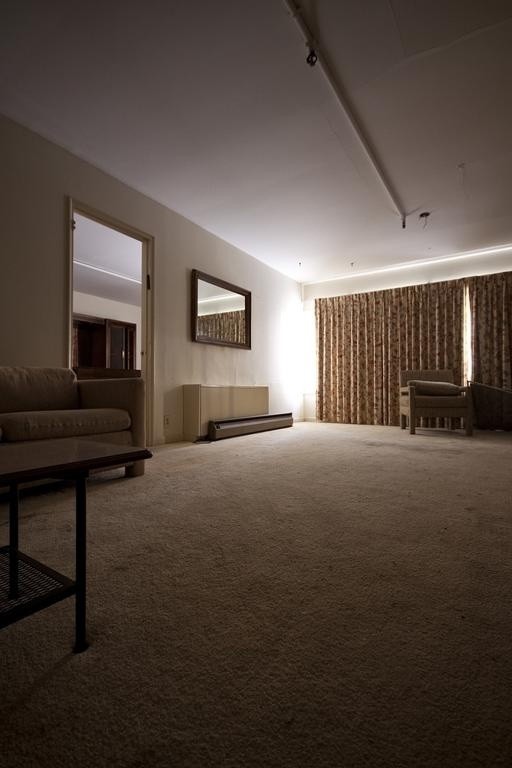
[191,267,252,351]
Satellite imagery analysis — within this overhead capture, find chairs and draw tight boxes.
[394,368,512,436]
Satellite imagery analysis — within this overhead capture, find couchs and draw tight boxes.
[1,363,149,481]
